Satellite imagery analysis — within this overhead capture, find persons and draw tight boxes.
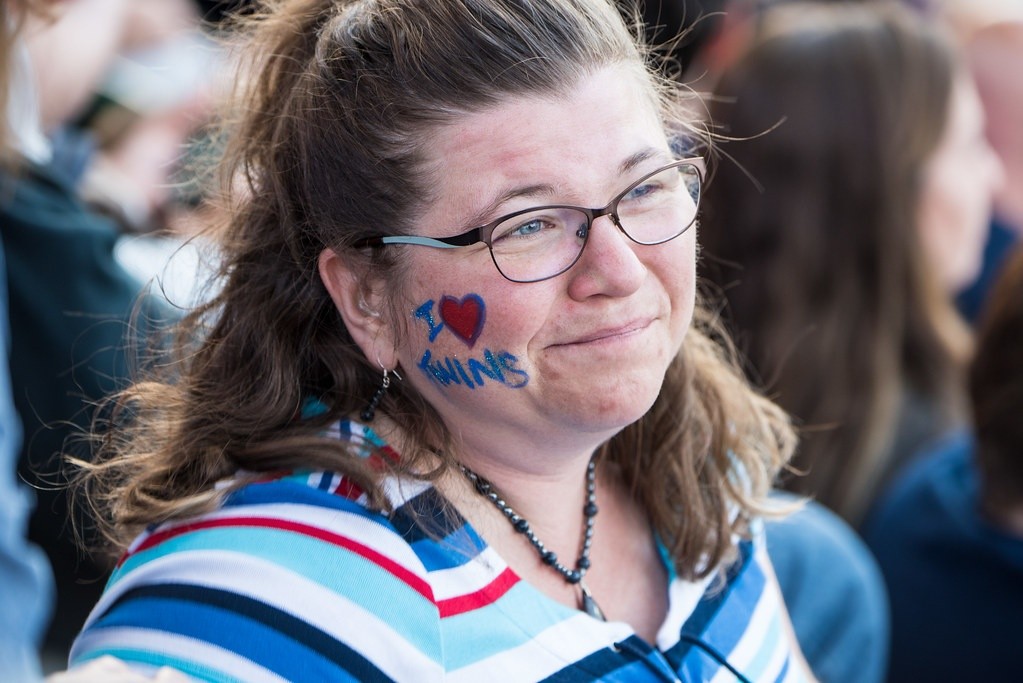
[68,1,819,683]
[694,1,1022,683]
[0,1,292,683]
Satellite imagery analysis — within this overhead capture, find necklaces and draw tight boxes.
[427,443,607,625]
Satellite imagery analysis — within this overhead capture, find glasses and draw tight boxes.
[347,155,706,282]
